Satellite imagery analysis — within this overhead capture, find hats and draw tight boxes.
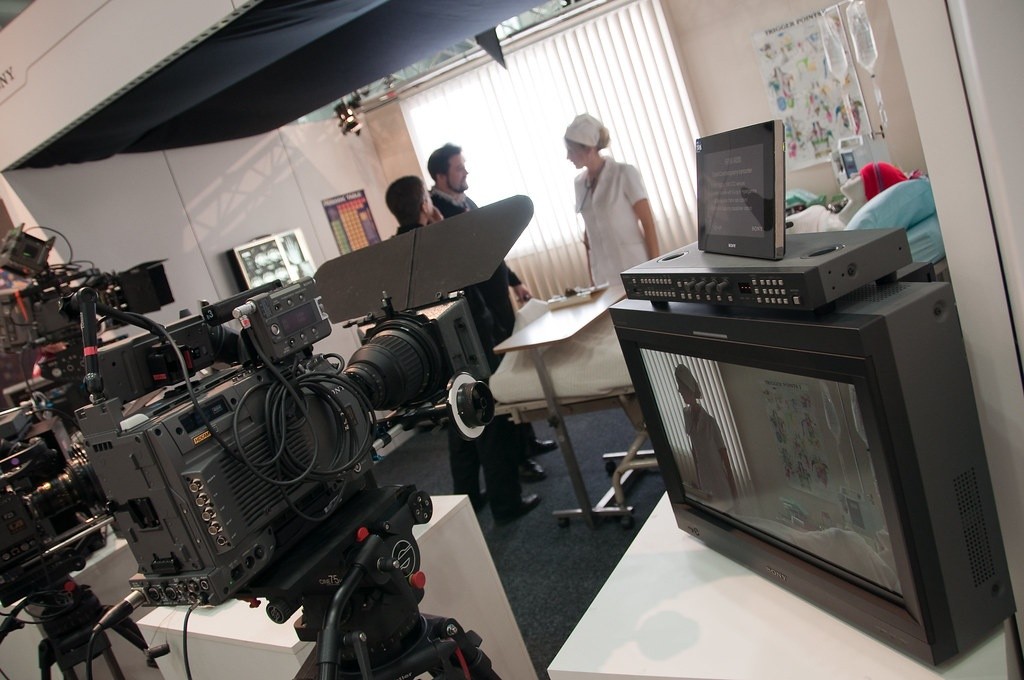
[566,114,602,147]
[860,161,921,200]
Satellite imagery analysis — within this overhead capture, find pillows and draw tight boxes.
[848,177,937,233]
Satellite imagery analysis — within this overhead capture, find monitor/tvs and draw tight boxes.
[605,262,1018,668]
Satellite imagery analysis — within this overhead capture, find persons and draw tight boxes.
[383,175,540,523]
[564,113,660,289]
[786,162,908,233]
[675,364,740,513]
[426,143,557,483]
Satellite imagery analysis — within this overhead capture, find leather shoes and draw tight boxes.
[527,438,556,454]
[474,489,488,510]
[517,458,546,484]
[493,494,541,526]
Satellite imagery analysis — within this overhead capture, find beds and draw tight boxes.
[486,217,945,529]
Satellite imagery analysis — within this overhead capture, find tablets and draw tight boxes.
[697,119,786,261]
